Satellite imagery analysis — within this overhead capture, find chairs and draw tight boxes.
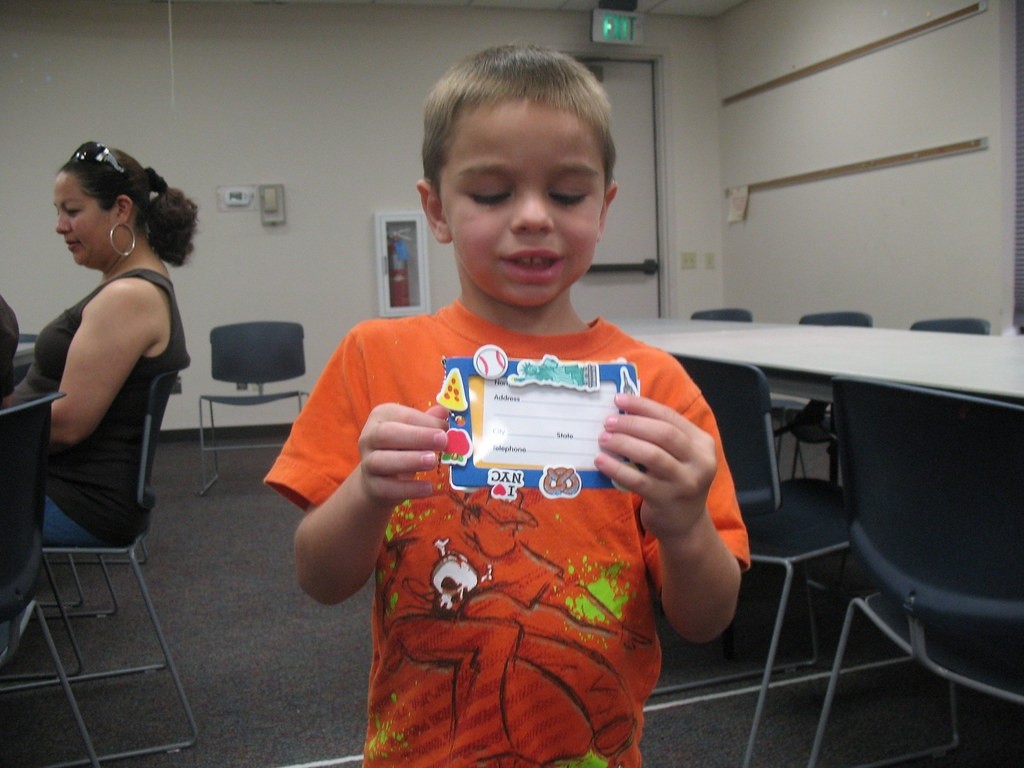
[1,370,199,768]
[199,322,310,496]
[650,310,1024,768]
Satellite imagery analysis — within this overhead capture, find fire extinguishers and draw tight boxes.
[387,234,410,307]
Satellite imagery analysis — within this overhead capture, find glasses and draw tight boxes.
[72,141,124,174]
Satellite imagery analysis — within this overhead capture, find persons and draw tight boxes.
[0,295,19,410]
[0,142,198,548]
[263,44,749,766]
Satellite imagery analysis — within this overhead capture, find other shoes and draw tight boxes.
[0,600,36,667]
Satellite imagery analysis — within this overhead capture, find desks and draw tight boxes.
[599,316,1024,404]
